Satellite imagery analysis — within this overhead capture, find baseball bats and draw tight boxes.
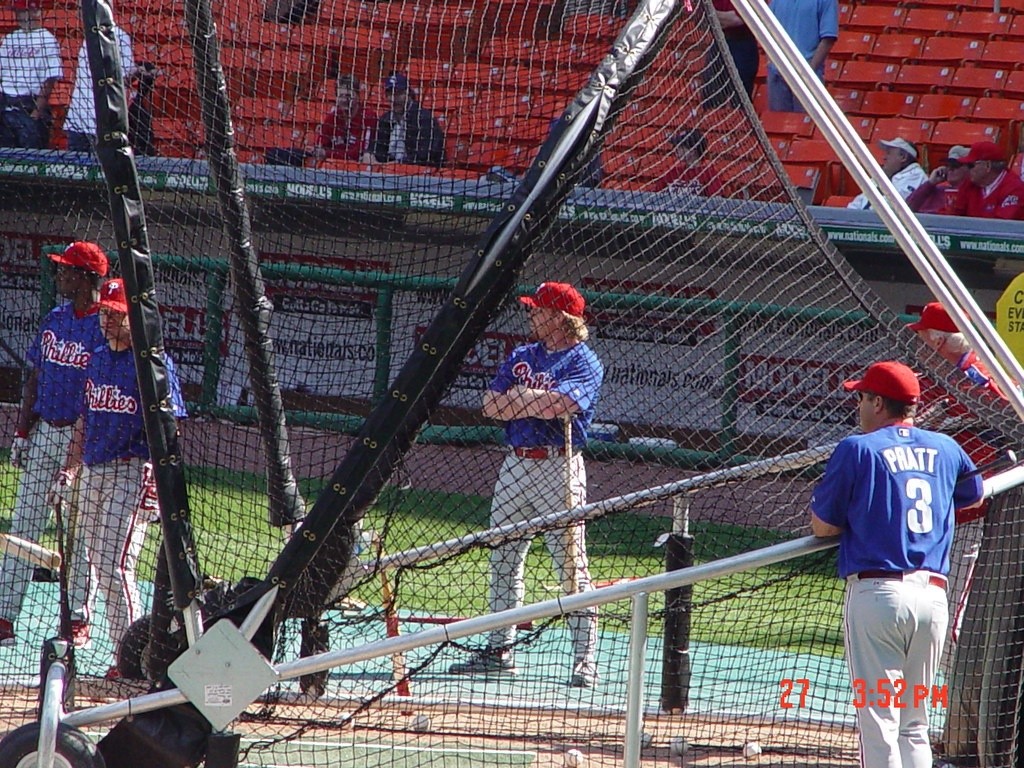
[55,502,75,671]
[0,533,62,569]
[371,536,414,717]
[561,412,580,595]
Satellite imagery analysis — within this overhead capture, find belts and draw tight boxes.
[37,412,75,429]
[857,568,947,593]
[513,445,569,460]
[113,452,135,462]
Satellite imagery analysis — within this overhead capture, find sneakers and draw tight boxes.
[570,654,599,689]
[0,618,16,647]
[448,642,520,676]
[57,620,91,648]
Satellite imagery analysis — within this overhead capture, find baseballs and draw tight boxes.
[641,732,651,748]
[671,737,688,755]
[566,749,583,767]
[336,712,354,729]
[411,712,428,733]
[743,742,762,760]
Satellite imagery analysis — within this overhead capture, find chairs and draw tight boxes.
[0,0,1023,216]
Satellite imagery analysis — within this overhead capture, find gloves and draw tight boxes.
[135,462,161,525]
[46,467,74,505]
[7,428,30,469]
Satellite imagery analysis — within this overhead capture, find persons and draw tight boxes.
[692,0,763,109]
[267,73,374,165]
[65,0,134,151]
[652,129,724,198]
[806,362,983,768]
[511,119,601,187]
[360,73,443,167]
[0,242,110,650]
[282,501,386,609]
[0,0,64,148]
[54,277,189,679]
[905,302,1023,752]
[448,282,598,689]
[848,136,1024,222]
[766,0,839,114]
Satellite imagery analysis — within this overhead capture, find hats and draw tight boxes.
[384,72,409,91]
[844,362,920,405]
[907,301,964,334]
[958,140,1008,164]
[520,281,586,318]
[95,278,128,314]
[878,137,917,160]
[938,143,971,163]
[46,241,108,278]
[667,128,705,152]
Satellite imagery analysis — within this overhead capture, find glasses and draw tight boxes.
[946,161,966,170]
